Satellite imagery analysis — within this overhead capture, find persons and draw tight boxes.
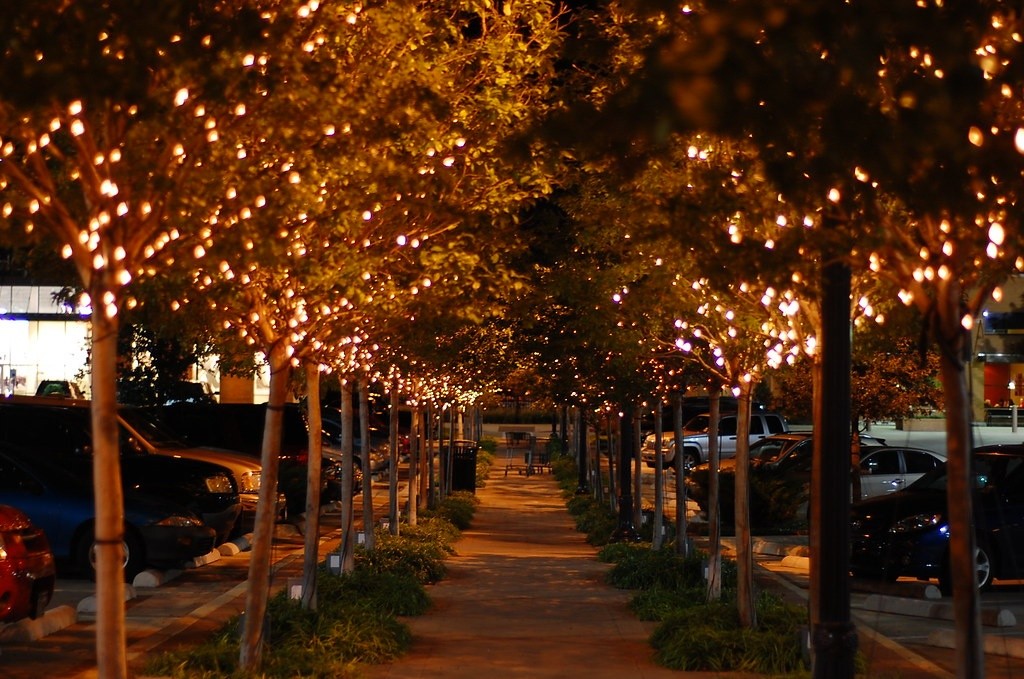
[984,398,1024,408]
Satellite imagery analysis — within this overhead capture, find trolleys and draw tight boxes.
[504,431,555,476]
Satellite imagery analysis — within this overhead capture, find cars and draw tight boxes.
[684,429,887,528]
[842,444,1024,597]
[768,446,949,534]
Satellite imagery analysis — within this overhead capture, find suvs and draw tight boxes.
[0,380,428,623]
[641,411,790,476]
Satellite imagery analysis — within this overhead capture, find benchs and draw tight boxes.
[505,464,552,476]
